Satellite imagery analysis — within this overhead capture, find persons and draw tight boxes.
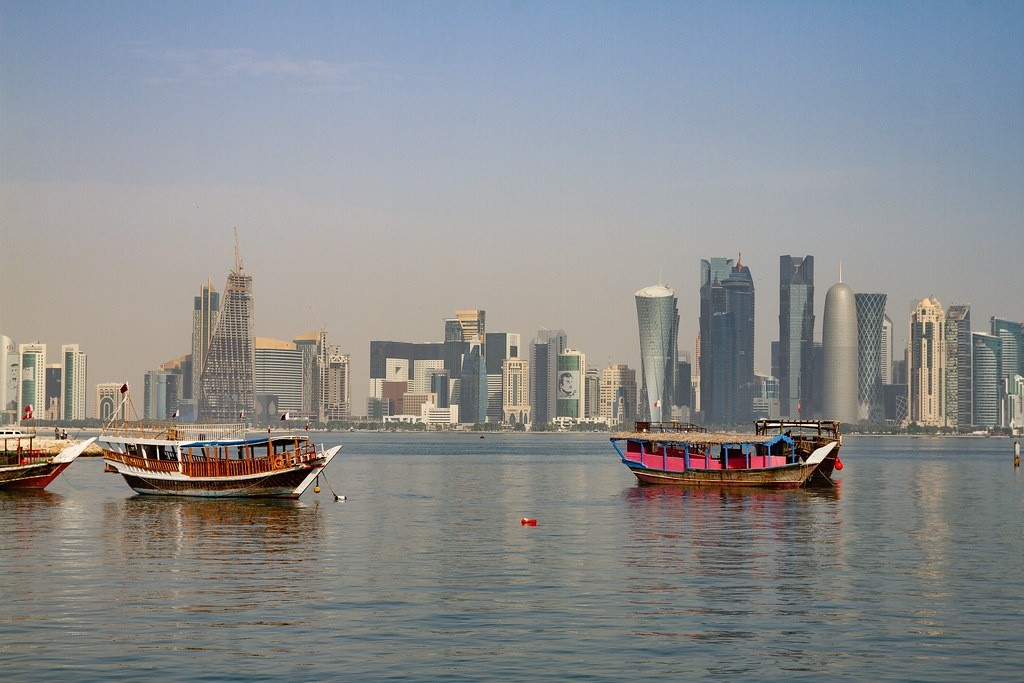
[784,430,792,438]
[63,429,67,439]
[55,428,59,439]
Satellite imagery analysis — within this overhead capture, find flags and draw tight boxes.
[172,409,179,418]
[798,402,801,412]
[280,412,289,420]
[306,422,309,432]
[23,404,33,420]
[120,382,128,394]
[654,400,661,408]
[240,411,243,418]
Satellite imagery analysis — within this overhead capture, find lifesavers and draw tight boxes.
[274,457,285,470]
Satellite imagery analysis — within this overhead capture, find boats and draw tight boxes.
[0,433,100,493]
[98,396,344,502]
[607,417,842,488]
